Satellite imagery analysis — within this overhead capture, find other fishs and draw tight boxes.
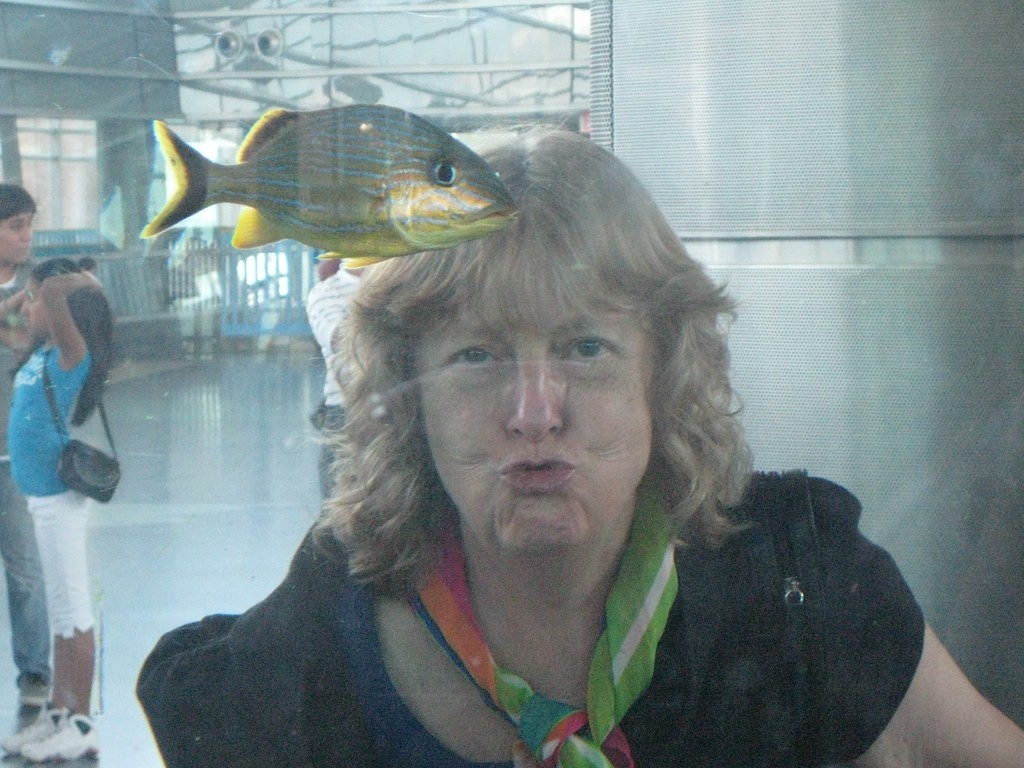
[133,103,518,270]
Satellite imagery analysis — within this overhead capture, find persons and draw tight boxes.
[306,262,386,508]
[0,184,55,708]
[0,255,114,763]
[136,124,1023,768]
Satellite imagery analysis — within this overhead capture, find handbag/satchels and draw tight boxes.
[57,440,121,502]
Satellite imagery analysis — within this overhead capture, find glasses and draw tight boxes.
[24,287,40,302]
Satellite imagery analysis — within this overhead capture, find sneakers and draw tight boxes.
[0,703,72,756]
[21,712,98,763]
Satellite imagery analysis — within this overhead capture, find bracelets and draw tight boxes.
[5,298,10,310]
